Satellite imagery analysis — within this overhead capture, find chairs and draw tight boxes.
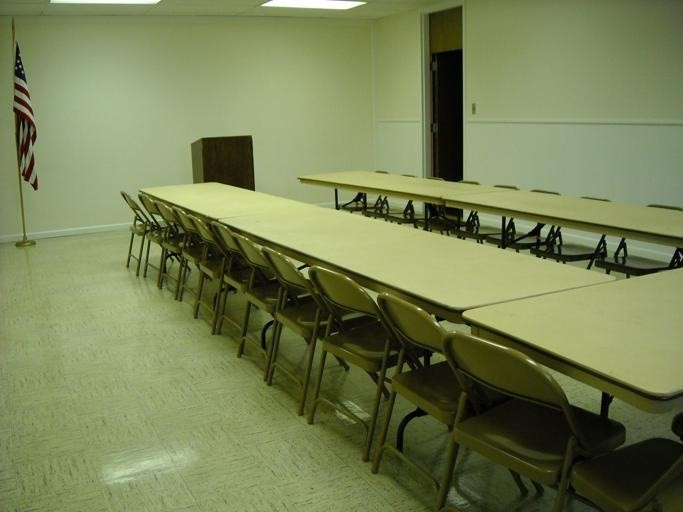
[300,169,683,282]
[364,294,546,511]
[436,329,626,512]
[559,434,683,511]
[119,182,378,417]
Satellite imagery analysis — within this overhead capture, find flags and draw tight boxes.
[12,38,39,191]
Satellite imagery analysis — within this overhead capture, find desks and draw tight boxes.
[464,265,682,436]
[219,205,618,454]
